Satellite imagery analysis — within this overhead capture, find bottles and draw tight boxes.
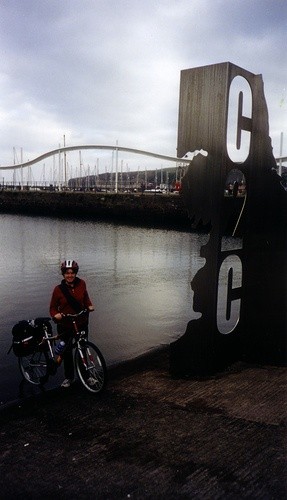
[53,341,65,355]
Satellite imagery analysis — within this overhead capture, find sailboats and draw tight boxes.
[0,135,187,194]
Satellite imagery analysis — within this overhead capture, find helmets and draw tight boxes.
[61,260,79,273]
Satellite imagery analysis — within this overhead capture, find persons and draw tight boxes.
[50,260,95,389]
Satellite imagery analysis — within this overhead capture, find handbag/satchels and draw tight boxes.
[12,320,36,356]
[33,316,53,352]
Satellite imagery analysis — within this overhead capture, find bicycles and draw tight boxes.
[17,308,108,394]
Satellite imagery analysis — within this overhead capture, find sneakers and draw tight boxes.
[61,379,71,388]
[85,377,98,386]
